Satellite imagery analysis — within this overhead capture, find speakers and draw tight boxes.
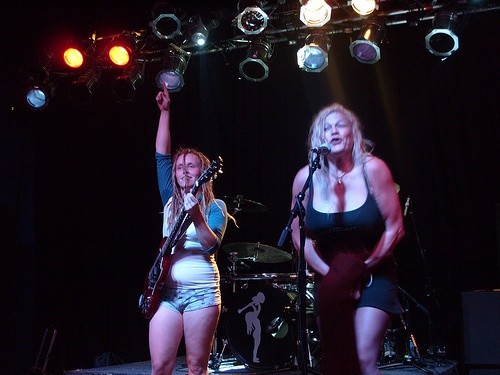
[459,289,500,367]
[465,366,500,375]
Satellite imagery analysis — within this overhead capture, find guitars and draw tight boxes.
[136,155,223,320]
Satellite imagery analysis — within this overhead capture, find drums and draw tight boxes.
[221,279,317,371]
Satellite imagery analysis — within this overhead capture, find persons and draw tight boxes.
[291,104,405,375]
[149,80,238,375]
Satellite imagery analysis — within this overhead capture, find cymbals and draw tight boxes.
[223,242,292,264]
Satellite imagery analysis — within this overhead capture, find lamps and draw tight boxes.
[63,37,95,70]
[152,0,183,40]
[155,42,190,93]
[349,16,388,64]
[25,75,54,111]
[425,9,461,59]
[238,32,274,83]
[235,0,269,35]
[297,27,333,73]
[106,29,146,69]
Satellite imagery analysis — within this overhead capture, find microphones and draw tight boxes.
[404,196,412,216]
[310,143,331,156]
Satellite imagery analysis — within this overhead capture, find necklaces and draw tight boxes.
[321,161,352,184]
